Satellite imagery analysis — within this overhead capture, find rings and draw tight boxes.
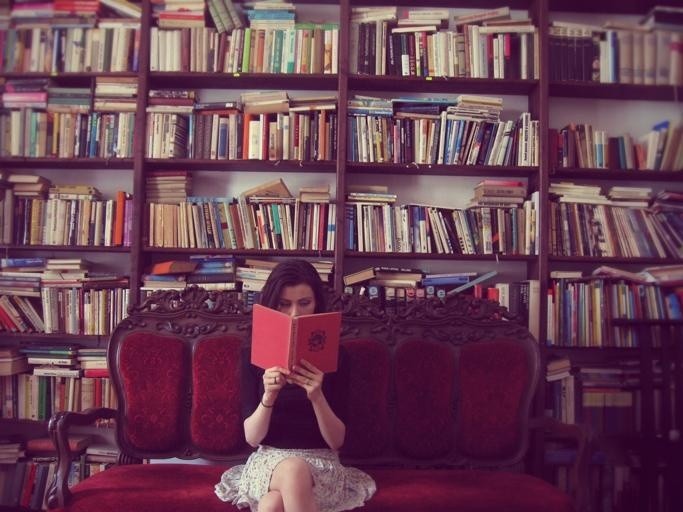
[306,379,310,384]
[274,377,277,384]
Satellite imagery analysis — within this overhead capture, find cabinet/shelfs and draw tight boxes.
[0,0,680,512]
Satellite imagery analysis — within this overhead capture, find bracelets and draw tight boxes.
[261,401,274,407]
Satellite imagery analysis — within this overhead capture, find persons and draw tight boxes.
[213,260,376,512]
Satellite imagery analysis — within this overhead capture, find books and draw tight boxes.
[1,436,121,510]
[341,7,540,339]
[141,1,338,308]
[251,304,342,376]
[547,5,683,512]
[1,0,141,430]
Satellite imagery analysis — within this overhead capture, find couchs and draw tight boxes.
[44,283,602,512]
